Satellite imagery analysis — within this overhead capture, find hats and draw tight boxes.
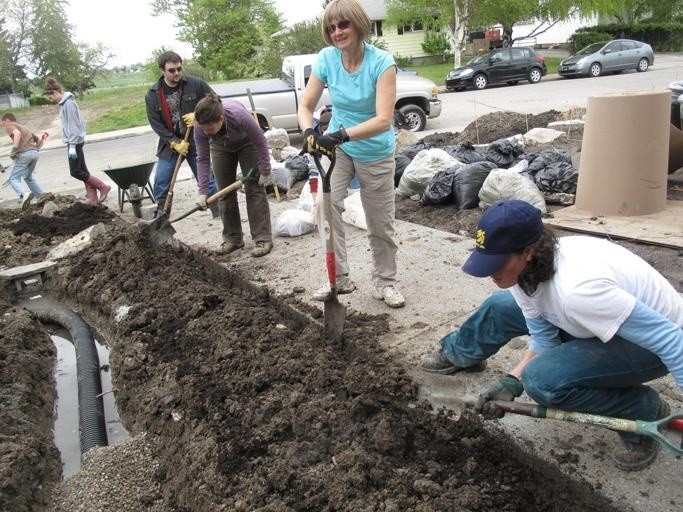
[458,199,544,278]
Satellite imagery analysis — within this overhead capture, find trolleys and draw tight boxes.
[100,157,155,218]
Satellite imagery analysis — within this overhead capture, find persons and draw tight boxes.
[297,0,408,308]
[143,51,248,222]
[40,77,110,208]
[307,102,360,202]
[0,113,44,210]
[192,92,272,257]
[419,199,683,472]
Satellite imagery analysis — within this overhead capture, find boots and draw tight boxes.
[79,181,99,207]
[207,196,222,218]
[88,174,112,204]
[154,197,172,219]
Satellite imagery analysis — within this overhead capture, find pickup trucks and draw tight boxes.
[204,53,442,139]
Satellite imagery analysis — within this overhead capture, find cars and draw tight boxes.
[446,47,547,92]
[558,38,654,77]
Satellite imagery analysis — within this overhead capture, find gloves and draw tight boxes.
[195,194,209,209]
[256,172,274,189]
[473,371,527,421]
[182,112,200,126]
[316,126,351,159]
[303,128,323,157]
[169,137,191,158]
[9,145,19,161]
[68,143,79,161]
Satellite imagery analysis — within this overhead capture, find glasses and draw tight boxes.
[163,64,183,74]
[325,17,352,35]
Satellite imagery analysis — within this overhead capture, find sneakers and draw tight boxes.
[419,341,488,374]
[250,238,274,258]
[20,191,35,211]
[612,389,672,473]
[314,272,357,302]
[212,235,246,254]
[370,277,406,308]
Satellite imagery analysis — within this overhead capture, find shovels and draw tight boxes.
[394,369,683,457]
[139,160,262,248]
[156,125,192,219]
[1,132,49,188]
[314,145,347,352]
